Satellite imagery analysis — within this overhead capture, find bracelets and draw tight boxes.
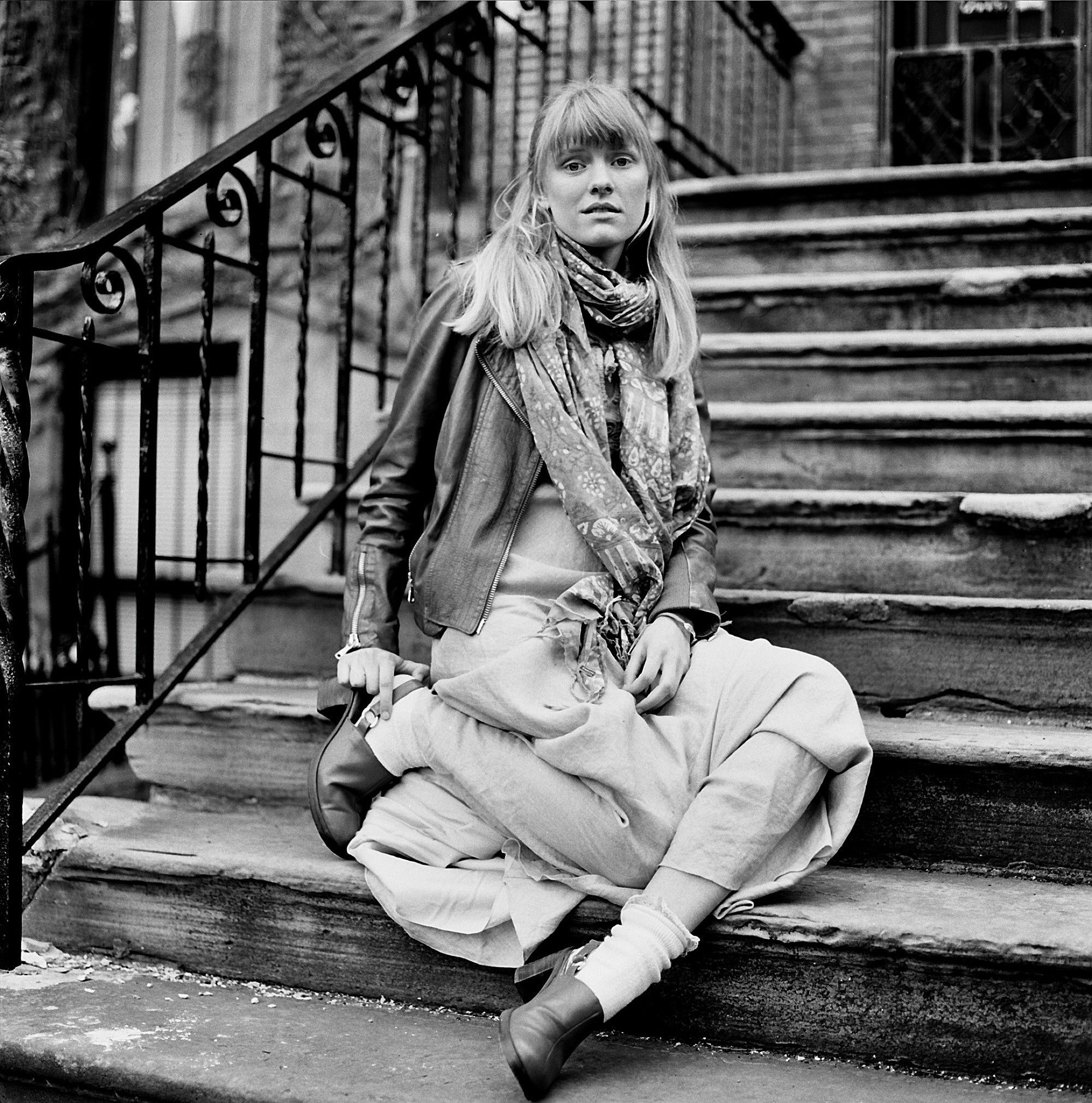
[656,612,697,645]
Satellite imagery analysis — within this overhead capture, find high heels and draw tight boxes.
[498,941,607,1101]
[307,677,424,861]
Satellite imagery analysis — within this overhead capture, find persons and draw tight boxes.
[310,77,873,1103]
